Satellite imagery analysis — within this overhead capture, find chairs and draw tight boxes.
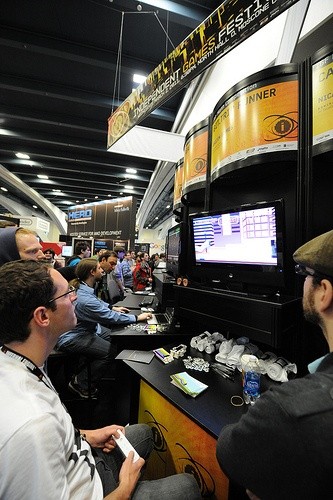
[45,348,92,404]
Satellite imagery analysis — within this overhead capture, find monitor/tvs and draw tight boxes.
[188,197,290,295]
[166,222,184,281]
[61,246,73,257]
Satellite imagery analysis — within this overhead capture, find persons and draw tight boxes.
[43,249,62,269]
[216,230,333,500]
[133,253,152,292]
[66,241,90,267]
[0,259,202,500]
[53,258,153,400]
[148,254,159,292]
[55,251,118,282]
[160,254,166,259]
[107,247,125,303]
[0,227,42,268]
[121,252,136,290]
[91,250,108,259]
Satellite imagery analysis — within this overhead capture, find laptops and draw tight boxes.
[146,305,176,325]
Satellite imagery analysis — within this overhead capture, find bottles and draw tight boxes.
[241,356,261,405]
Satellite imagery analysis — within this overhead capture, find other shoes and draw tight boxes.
[68,374,98,401]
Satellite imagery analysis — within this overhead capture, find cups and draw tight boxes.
[241,354,258,385]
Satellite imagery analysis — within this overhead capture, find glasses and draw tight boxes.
[294,264,321,286]
[28,284,75,321]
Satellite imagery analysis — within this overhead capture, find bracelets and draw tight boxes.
[135,315,138,322]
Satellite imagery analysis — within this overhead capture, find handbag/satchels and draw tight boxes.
[101,290,109,302]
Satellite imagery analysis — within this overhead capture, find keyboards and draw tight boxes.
[139,296,154,306]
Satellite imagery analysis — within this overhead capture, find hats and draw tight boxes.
[292,229,333,273]
